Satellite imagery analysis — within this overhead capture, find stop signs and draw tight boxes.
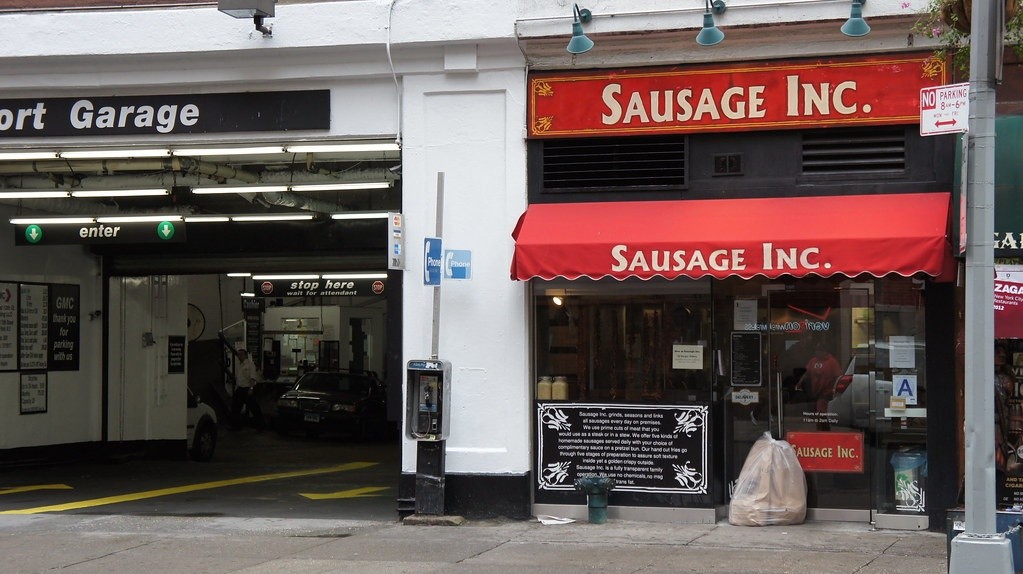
[262,281,273,295]
[372,281,384,295]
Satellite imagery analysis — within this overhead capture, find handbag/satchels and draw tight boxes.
[728,431,808,526]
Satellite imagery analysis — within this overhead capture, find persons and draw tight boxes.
[226,349,264,432]
[796,341,839,430]
[765,354,813,417]
[750,402,766,426]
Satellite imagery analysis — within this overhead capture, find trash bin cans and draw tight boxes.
[890,446,929,517]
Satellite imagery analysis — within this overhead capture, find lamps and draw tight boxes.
[217,0,276,38]
[696,0,727,47]
[840,0,873,37]
[566,2,594,53]
[0,138,404,300]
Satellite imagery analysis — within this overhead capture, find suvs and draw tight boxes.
[827,339,928,447]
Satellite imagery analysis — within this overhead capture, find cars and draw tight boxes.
[273,368,389,442]
[186,386,219,464]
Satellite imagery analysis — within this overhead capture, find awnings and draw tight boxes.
[510,192,957,285]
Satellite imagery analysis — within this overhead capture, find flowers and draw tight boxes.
[899,0,1023,80]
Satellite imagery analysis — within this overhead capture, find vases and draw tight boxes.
[937,0,1021,35]
